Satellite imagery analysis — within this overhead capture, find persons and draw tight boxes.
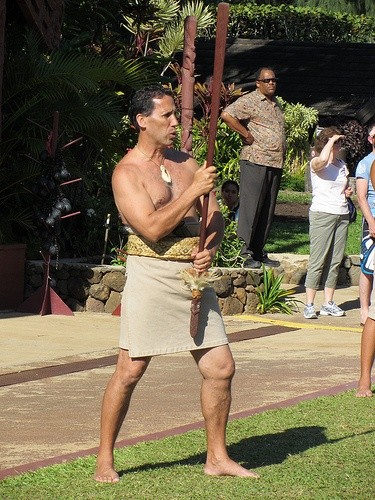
[91,81,261,484]
[355,126,375,399]
[221,66,287,269]
[219,179,240,223]
[303,127,354,319]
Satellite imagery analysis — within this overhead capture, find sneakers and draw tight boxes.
[303,304,318,319]
[320,302,345,316]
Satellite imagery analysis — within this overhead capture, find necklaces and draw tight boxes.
[137,144,172,184]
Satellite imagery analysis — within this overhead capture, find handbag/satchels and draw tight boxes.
[346,196,358,222]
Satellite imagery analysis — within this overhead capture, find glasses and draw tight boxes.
[257,78,277,83]
[334,138,344,144]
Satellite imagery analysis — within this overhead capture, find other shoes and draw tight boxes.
[253,255,280,267]
[237,258,261,269]
